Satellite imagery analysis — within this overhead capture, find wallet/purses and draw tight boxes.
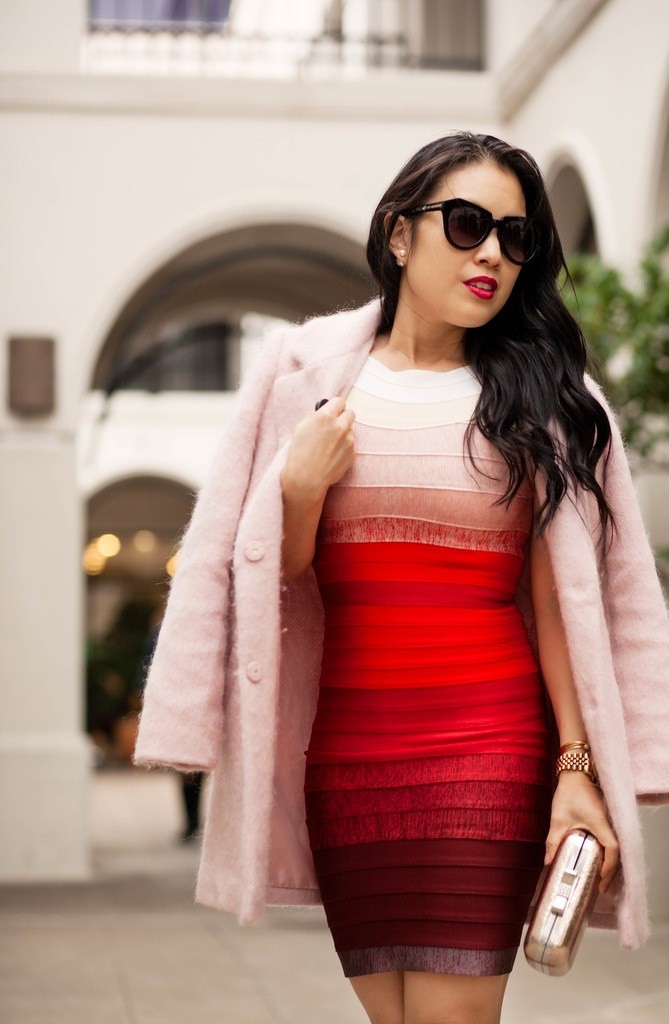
[523,831,600,977]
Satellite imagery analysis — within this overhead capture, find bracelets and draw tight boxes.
[556,753,596,781]
[561,740,591,753]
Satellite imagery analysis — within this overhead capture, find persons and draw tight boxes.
[134,134,669,1024]
[119,605,202,844]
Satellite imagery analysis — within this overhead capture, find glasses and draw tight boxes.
[410,198,544,265]
